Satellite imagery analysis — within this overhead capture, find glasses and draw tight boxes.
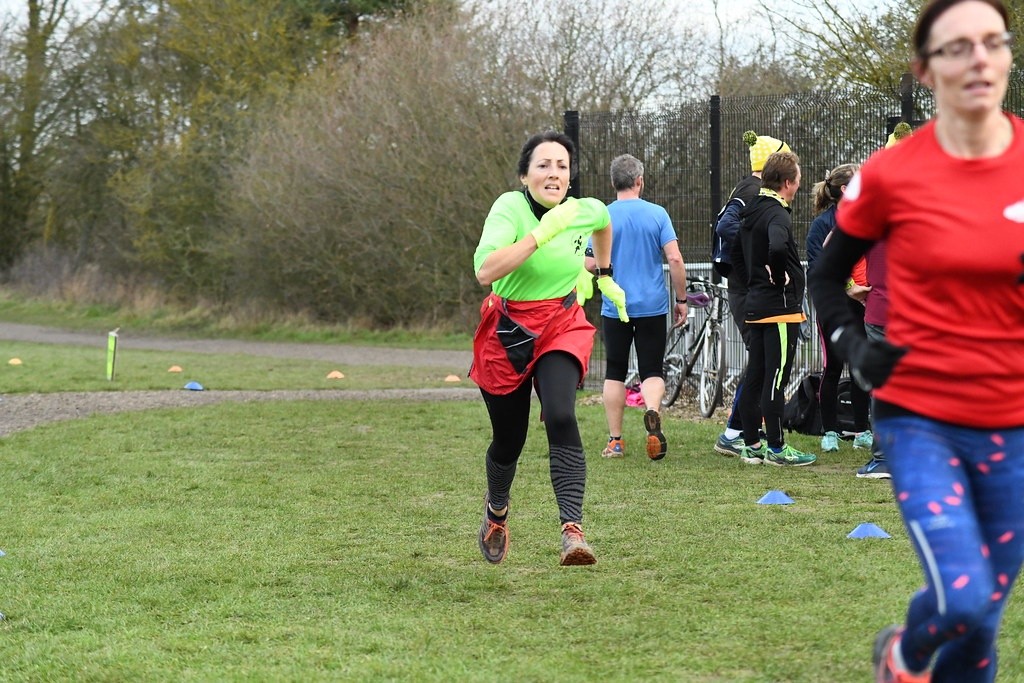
[922,32,1014,63]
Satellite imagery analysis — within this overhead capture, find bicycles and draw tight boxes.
[661,276,732,418]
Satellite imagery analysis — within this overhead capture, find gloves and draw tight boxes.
[596,276,630,323]
[531,199,595,248]
[834,325,907,387]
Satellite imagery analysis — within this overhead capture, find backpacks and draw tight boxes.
[835,377,873,440]
[783,371,826,436]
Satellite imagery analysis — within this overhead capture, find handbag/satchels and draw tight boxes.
[626,383,644,407]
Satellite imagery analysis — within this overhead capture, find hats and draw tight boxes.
[743,130,791,172]
[885,122,914,149]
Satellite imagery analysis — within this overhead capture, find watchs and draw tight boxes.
[595,264,613,277]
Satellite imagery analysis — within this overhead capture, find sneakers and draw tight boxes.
[872,625,930,683]
[559,520,597,567]
[821,431,844,452]
[853,430,874,451]
[741,443,768,465]
[713,432,745,458]
[763,444,816,467]
[856,459,892,479]
[644,410,667,460]
[758,430,767,441]
[601,439,624,458]
[478,492,509,564]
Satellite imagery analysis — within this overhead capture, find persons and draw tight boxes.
[807,121,913,480]
[466,130,613,565]
[586,154,688,461]
[806,0,1024,683]
[712,131,818,466]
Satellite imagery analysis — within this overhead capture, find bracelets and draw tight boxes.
[675,298,687,303]
